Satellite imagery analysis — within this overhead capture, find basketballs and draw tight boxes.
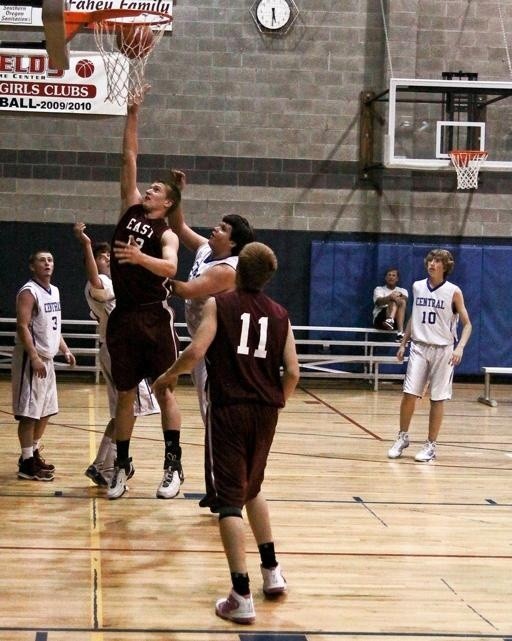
[76,59,94,77]
[116,25,154,58]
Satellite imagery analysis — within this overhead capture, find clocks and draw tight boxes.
[249,0,300,36]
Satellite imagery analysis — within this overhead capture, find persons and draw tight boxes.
[372,266,411,342]
[388,248,472,460]
[106,84,184,501]
[150,241,299,624]
[72,220,162,490]
[168,168,255,513]
[11,248,77,482]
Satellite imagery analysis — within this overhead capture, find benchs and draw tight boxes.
[476,366,512,408]
[0,313,412,395]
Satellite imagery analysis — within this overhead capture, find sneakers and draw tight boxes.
[390,429,439,462]
[85,459,135,497]
[157,464,185,497]
[215,590,256,618]
[18,450,55,482]
[260,564,288,594]
[383,317,404,341]
